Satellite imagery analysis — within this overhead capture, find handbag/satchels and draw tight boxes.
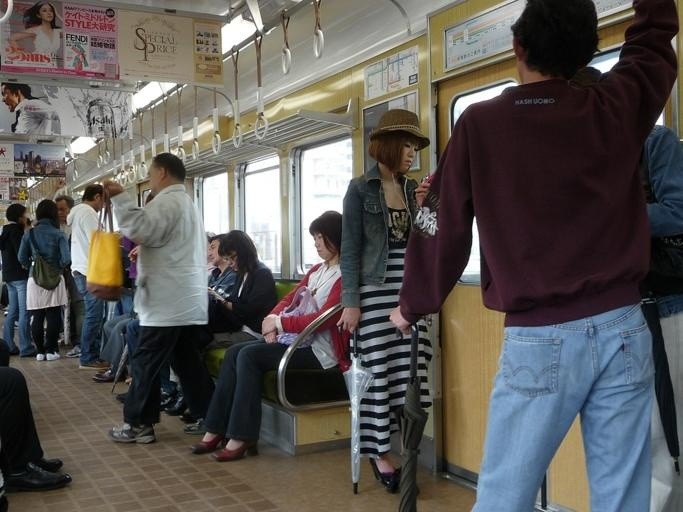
[642,235,682,296]
[276,285,319,349]
[86,231,124,301]
[32,253,60,290]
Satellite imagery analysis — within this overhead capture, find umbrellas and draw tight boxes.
[394,321,429,511]
[336,323,374,496]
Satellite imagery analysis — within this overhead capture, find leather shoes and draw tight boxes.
[160,391,206,434]
[369,458,396,486]
[5,458,72,492]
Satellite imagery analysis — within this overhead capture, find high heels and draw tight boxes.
[191,430,228,454]
[212,439,258,462]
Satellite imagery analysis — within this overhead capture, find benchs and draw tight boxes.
[199,278,355,456]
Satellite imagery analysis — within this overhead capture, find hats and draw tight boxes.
[369,109,430,151]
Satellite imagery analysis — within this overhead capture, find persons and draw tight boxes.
[636,125,682,512]
[93,230,276,421]
[1,185,156,367]
[0,82,59,134]
[387,0,680,511]
[102,152,215,445]
[6,0,63,69]
[1,340,71,491]
[191,211,343,460]
[336,109,434,494]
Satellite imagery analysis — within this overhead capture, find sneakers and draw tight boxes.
[65,344,128,382]
[37,351,59,360]
[110,425,155,444]
